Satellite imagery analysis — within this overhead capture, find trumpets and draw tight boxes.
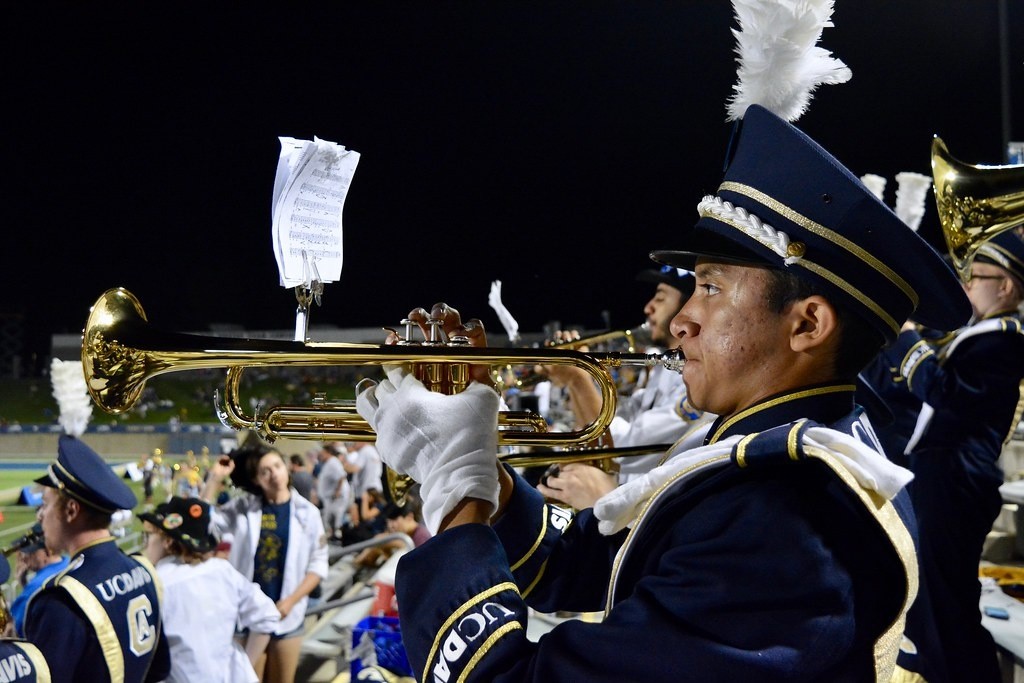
[926,127,1022,291]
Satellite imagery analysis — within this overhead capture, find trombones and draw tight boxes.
[78,277,732,512]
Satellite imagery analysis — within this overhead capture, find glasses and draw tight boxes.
[141,530,161,544]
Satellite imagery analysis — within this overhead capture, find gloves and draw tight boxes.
[355,363,501,537]
[593,427,915,536]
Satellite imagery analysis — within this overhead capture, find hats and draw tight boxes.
[974,230,1024,286]
[33,358,137,516]
[137,495,218,552]
[648,250,697,293]
[0,553,10,585]
[647,0,973,350]
[11,523,45,553]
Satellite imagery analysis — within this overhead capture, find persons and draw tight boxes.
[0,172,1024,683]
[357,2,975,683]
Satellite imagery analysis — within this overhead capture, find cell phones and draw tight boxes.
[984,607,1009,620]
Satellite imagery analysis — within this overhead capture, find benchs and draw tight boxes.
[302,583,365,658]
